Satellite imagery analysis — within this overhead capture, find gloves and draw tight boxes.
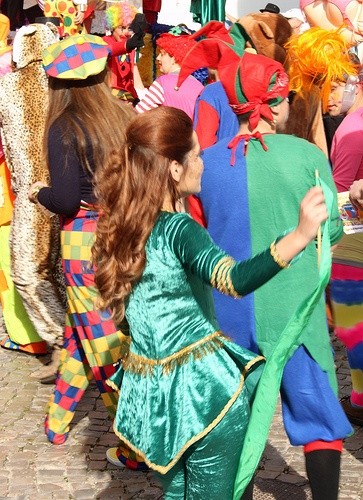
[126,28,145,51]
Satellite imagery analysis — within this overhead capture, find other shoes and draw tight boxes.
[29,344,62,383]
[0,334,47,355]
[339,394,363,428]
[105,447,126,468]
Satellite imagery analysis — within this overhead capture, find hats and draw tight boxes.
[42,33,112,89]
[34,16,64,41]
[143,0,304,129]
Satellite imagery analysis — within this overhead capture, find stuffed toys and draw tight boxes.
[237,5,292,71]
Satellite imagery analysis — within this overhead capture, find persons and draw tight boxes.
[29,34,153,469]
[92,106,328,500]
[188,54,355,500]
[0,1,363,424]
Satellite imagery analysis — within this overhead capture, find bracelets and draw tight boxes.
[32,185,44,202]
